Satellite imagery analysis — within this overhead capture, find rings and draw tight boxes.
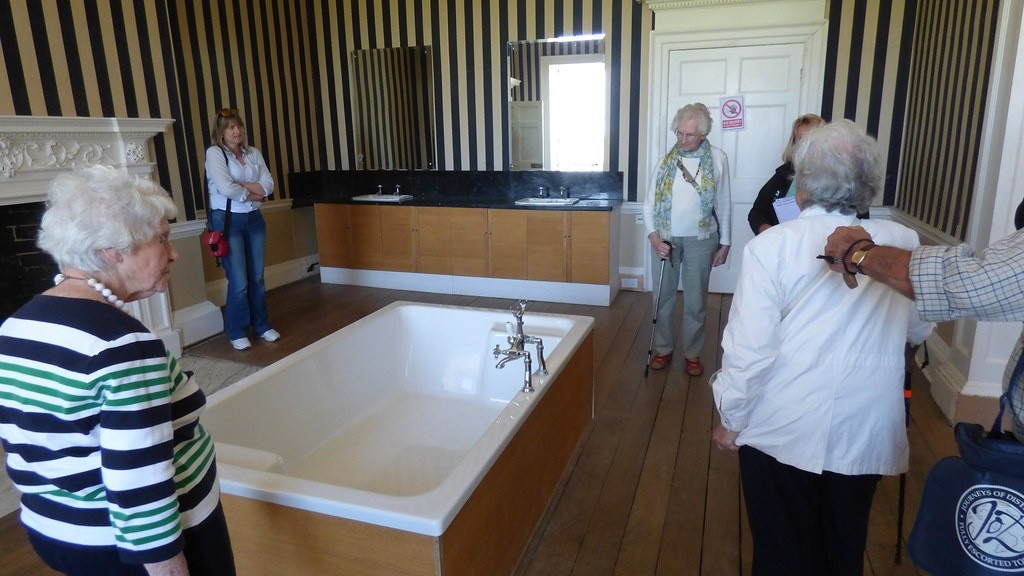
[660,252,663,255]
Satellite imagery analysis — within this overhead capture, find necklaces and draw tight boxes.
[53,273,135,315]
[680,155,701,183]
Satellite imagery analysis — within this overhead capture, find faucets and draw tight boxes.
[394,185,401,196]
[376,185,383,196]
[506,335,548,376]
[559,186,566,198]
[493,345,535,393]
[538,186,544,199]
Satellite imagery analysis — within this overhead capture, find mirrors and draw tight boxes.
[508,34,610,173]
[349,44,439,174]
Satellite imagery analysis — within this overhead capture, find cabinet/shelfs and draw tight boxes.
[448,205,528,299]
[316,203,381,288]
[377,204,454,296]
[524,205,621,308]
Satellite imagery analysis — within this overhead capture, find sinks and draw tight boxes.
[352,194,413,202]
[515,197,580,205]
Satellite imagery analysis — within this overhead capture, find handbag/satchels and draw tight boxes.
[204,231,228,268]
[907,418,1024,574]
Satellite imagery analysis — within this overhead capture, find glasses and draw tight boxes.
[674,129,699,140]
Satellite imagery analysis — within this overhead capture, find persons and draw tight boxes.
[747,113,824,236]
[640,102,733,376]
[824,223,1024,438]
[710,120,933,576]
[205,108,279,352]
[0,161,237,576]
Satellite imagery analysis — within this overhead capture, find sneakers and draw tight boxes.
[258,328,281,343]
[685,356,704,376]
[229,337,252,351]
[651,353,672,369]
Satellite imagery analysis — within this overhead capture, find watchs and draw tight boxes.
[850,243,877,276]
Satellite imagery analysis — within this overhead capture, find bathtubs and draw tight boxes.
[197,300,597,576]
